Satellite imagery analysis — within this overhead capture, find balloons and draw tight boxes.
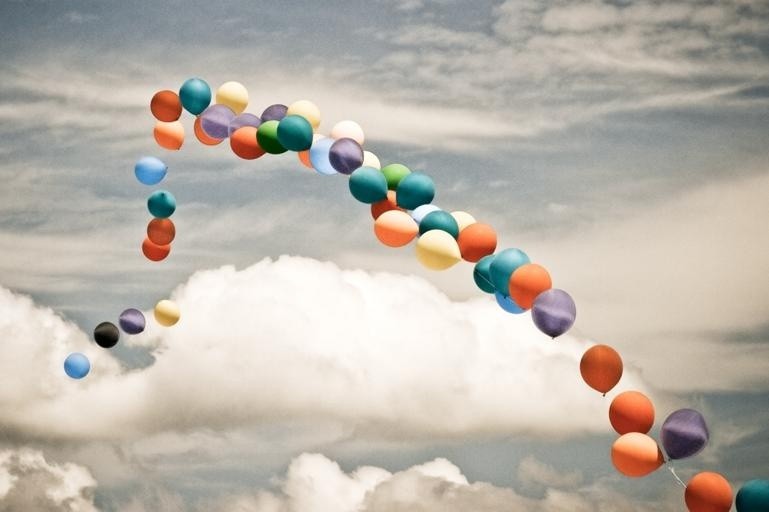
[735,479,767,512]
[473,248,577,339]
[660,408,709,460]
[150,78,320,160]
[136,157,168,186]
[580,344,623,397]
[64,353,90,379]
[154,299,180,327]
[147,190,177,218]
[94,321,120,347]
[147,217,176,246]
[142,236,171,262]
[609,391,655,436]
[120,309,146,334]
[611,432,665,478]
[299,121,497,270]
[684,472,734,512]
